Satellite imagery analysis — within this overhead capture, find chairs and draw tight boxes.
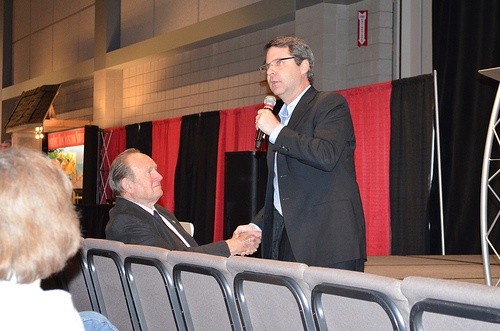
[58,234,500,331]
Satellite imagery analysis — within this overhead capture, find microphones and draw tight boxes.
[255,95,276,147]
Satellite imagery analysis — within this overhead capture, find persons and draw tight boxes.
[232,34,367,273]
[105,148,262,258]
[0,146,120,331]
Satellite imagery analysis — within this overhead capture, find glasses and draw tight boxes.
[260,57,294,72]
